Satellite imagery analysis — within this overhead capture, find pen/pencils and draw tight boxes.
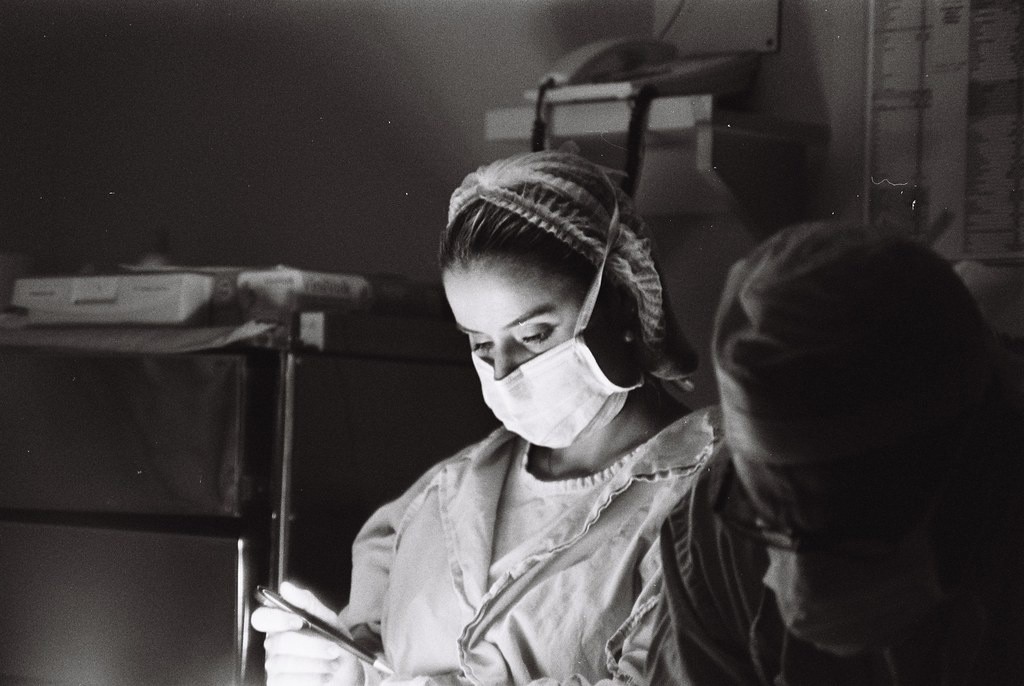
[256,584,397,676]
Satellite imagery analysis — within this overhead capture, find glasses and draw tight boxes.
[714,457,924,554]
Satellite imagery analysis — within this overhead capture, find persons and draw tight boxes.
[611,220,1024,686]
[250,152,722,686]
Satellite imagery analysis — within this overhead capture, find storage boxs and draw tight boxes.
[10,267,371,328]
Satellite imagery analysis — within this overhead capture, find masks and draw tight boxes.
[761,545,937,657]
[472,260,644,449]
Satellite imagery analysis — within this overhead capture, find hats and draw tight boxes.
[710,224,999,465]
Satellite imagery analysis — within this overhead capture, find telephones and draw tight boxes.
[523,37,763,104]
